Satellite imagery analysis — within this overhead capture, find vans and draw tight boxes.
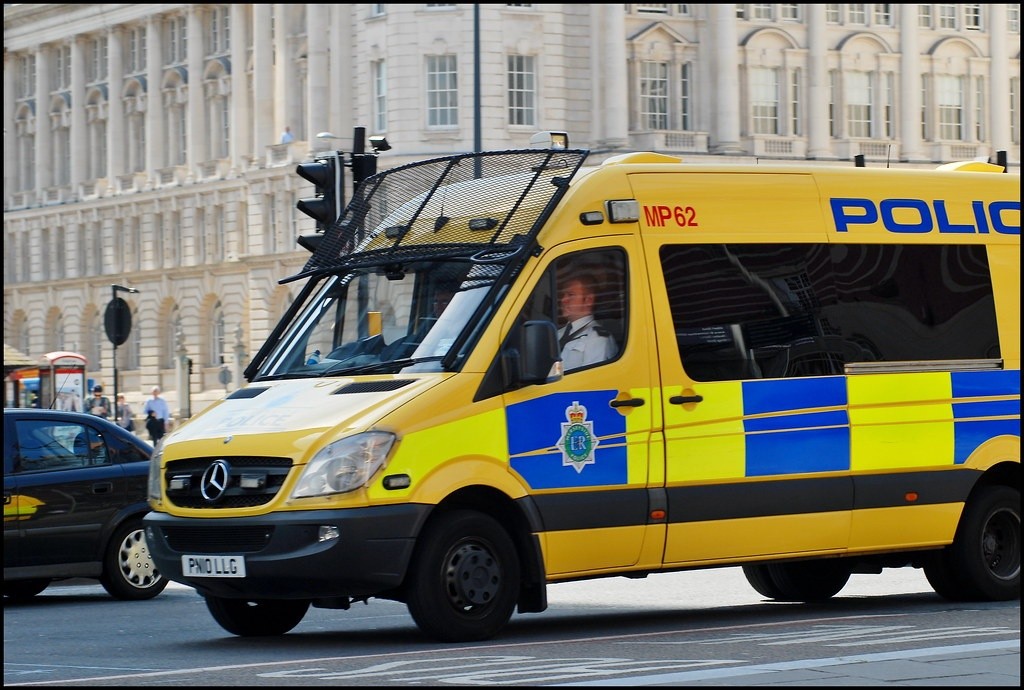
[141,142,1023,645]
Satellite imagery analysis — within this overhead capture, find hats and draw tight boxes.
[90,386,102,392]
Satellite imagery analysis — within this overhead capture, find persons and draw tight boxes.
[433,281,450,318]
[31,385,169,446]
[551,278,618,370]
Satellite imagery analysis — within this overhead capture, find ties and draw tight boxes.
[559,322,572,351]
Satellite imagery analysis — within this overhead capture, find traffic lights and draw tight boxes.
[293,151,344,267]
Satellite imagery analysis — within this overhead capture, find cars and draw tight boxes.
[4,406,174,602]
